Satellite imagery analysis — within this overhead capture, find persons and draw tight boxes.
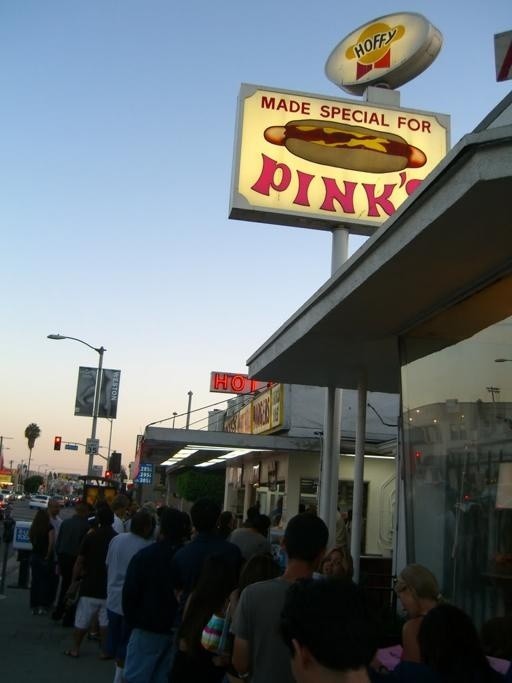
[29,493,493,683]
[77,368,114,417]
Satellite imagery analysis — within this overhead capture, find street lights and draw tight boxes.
[187,389,193,428]
[48,333,106,484]
[172,412,177,428]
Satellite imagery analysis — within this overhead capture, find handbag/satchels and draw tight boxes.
[201,614,232,657]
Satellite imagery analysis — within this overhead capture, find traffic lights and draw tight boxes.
[54,435,61,450]
[105,470,111,479]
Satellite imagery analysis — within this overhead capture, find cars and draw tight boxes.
[0,488,83,510]
